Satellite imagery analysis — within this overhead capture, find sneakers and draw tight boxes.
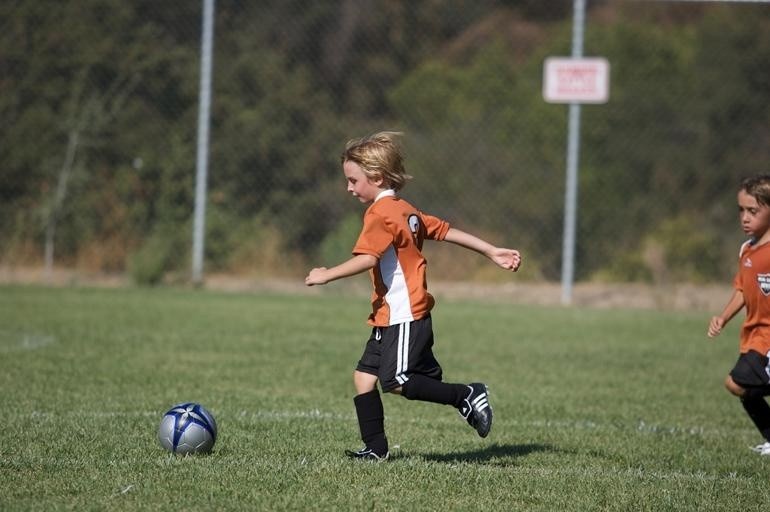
[752,442,770,454]
[457,383,493,438]
[345,448,390,462]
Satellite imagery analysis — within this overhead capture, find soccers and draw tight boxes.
[157,402,217,456]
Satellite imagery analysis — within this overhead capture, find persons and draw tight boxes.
[306,129,522,465]
[708,173,770,453]
